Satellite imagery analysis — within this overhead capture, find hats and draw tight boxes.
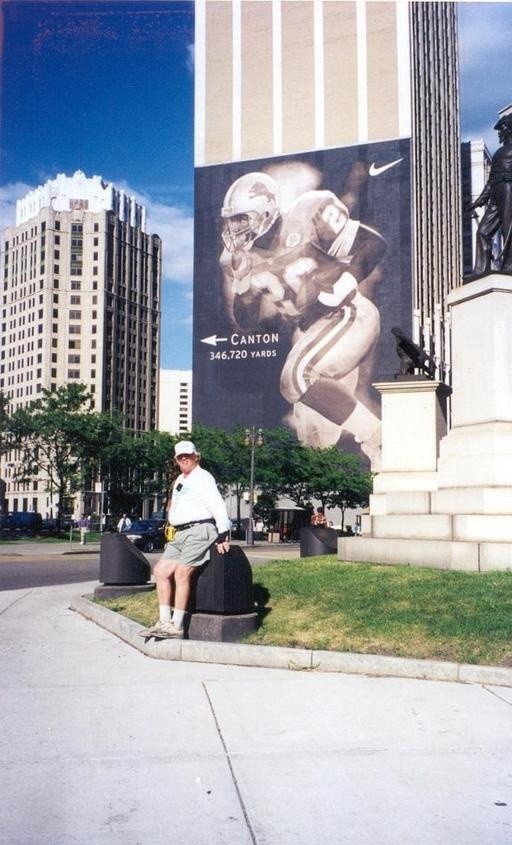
[174,440,195,459]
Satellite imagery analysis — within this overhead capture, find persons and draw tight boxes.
[311,507,327,528]
[462,113,512,281]
[139,440,231,638]
[79,514,89,545]
[219,171,388,473]
[328,520,335,528]
[164,521,176,541]
[117,513,132,533]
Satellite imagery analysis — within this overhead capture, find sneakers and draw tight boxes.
[149,619,184,638]
[138,620,164,637]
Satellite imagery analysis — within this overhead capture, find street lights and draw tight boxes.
[245,426,266,544]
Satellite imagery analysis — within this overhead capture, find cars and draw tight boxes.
[0,511,77,531]
[88,515,109,531]
[120,518,169,553]
[230,517,269,541]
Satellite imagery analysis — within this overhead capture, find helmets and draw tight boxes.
[219,171,286,258]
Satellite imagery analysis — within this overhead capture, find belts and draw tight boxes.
[174,519,215,532]
